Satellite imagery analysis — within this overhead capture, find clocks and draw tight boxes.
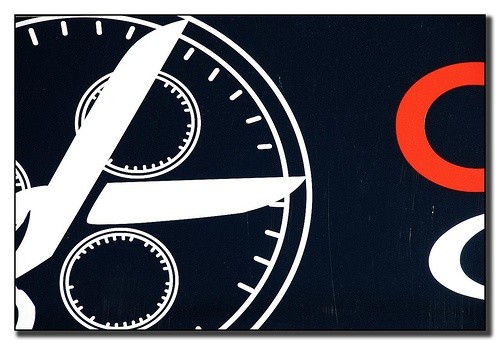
[14,14,314,331]
